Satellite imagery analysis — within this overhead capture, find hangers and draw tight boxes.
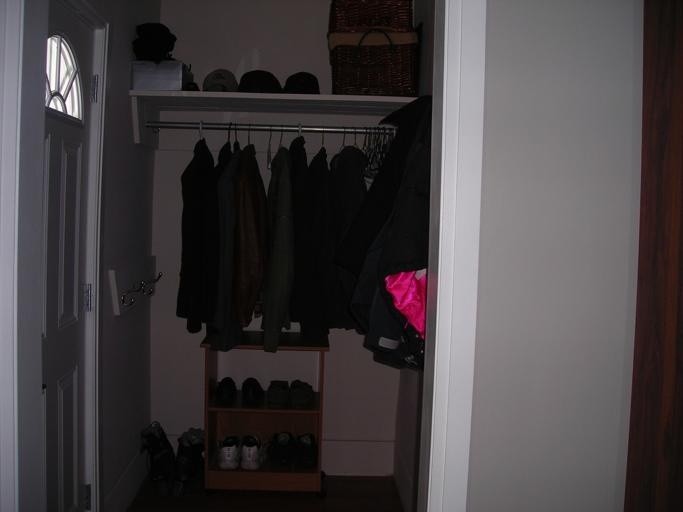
[195,121,395,184]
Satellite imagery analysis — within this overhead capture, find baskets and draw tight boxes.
[327,28,420,95]
[329,2,415,35]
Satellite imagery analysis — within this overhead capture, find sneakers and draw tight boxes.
[242,434,261,469]
[212,377,314,409]
[271,431,295,467]
[288,433,316,469]
[219,435,240,469]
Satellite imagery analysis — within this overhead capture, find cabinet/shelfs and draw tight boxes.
[200,331,330,493]
[133,61,194,92]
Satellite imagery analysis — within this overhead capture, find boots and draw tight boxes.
[174,428,203,486]
[138,422,175,482]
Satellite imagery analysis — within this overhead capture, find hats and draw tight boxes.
[279,72,319,94]
[237,71,284,93]
[202,70,238,92]
